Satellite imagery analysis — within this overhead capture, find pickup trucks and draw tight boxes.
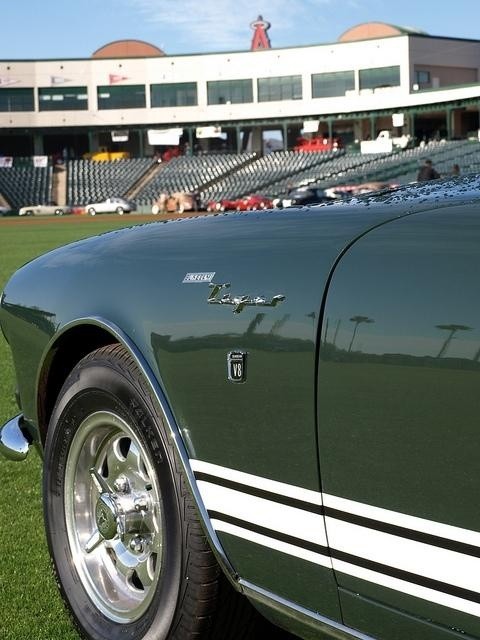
[82,145,131,160]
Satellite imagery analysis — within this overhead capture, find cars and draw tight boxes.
[207,192,273,213]
[0,205,13,217]
[294,134,345,152]
[330,182,389,200]
[151,191,193,215]
[19,201,72,216]
[84,196,136,216]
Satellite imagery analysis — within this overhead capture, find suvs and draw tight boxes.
[272,186,325,209]
[0,172,480,640]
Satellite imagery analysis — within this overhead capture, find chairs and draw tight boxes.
[1,139,480,214]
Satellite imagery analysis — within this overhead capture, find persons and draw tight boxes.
[154,142,189,161]
[417,160,440,182]
[447,164,460,178]
